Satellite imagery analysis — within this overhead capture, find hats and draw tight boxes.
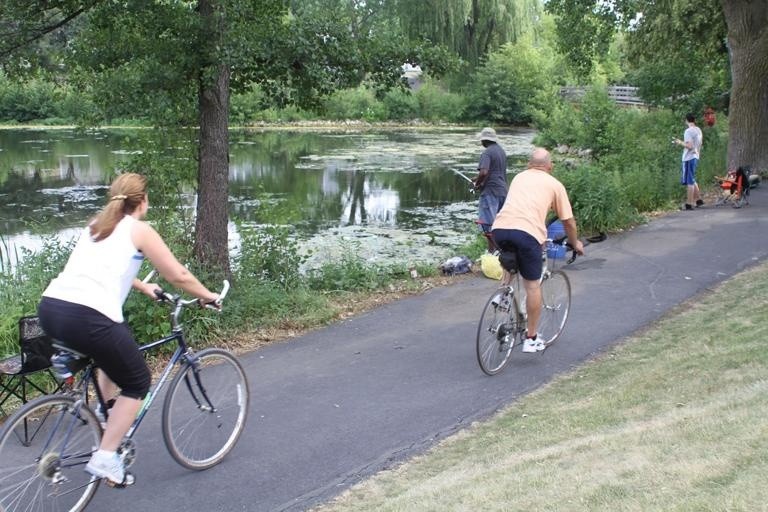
[477,128,497,142]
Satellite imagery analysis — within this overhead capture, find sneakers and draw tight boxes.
[95,404,113,430]
[492,294,511,312]
[679,203,694,210]
[522,332,545,353]
[693,200,703,207]
[85,450,134,486]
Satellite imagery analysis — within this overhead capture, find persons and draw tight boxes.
[470,127,508,257]
[38,173,222,487]
[704,107,715,127]
[491,149,584,353]
[673,112,703,209]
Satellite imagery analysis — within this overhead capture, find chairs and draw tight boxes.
[474,216,503,256]
[716,166,749,208]
[0,316,89,446]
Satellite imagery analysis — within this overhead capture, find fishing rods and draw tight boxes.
[450,164,475,202]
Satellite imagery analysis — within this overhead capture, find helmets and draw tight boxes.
[481,255,503,280]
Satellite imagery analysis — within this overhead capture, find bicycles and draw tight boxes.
[0,267,252,511]
[474,232,575,376]
